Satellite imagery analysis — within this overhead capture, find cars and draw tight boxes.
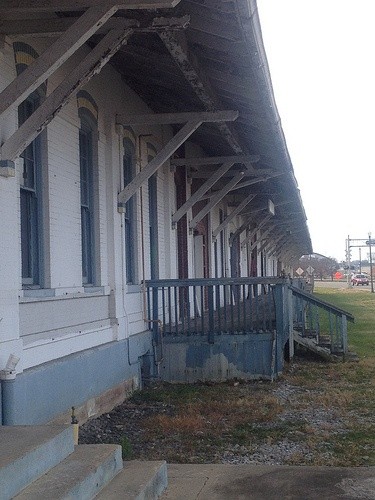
[351,274,369,286]
